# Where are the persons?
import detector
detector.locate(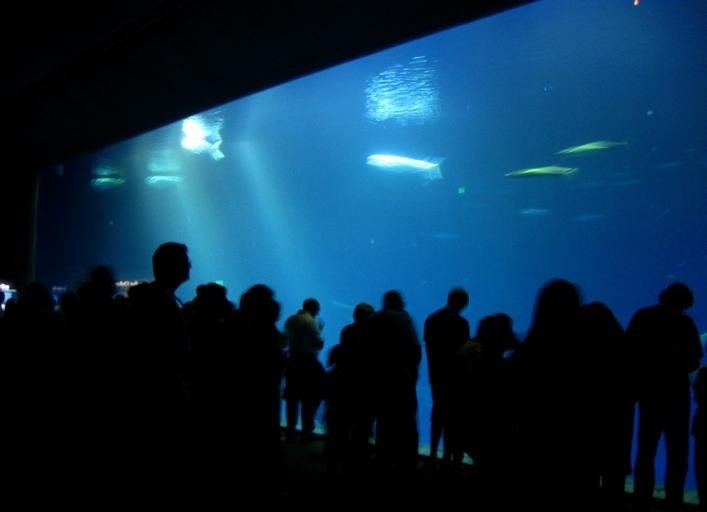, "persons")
[1,242,706,512]
